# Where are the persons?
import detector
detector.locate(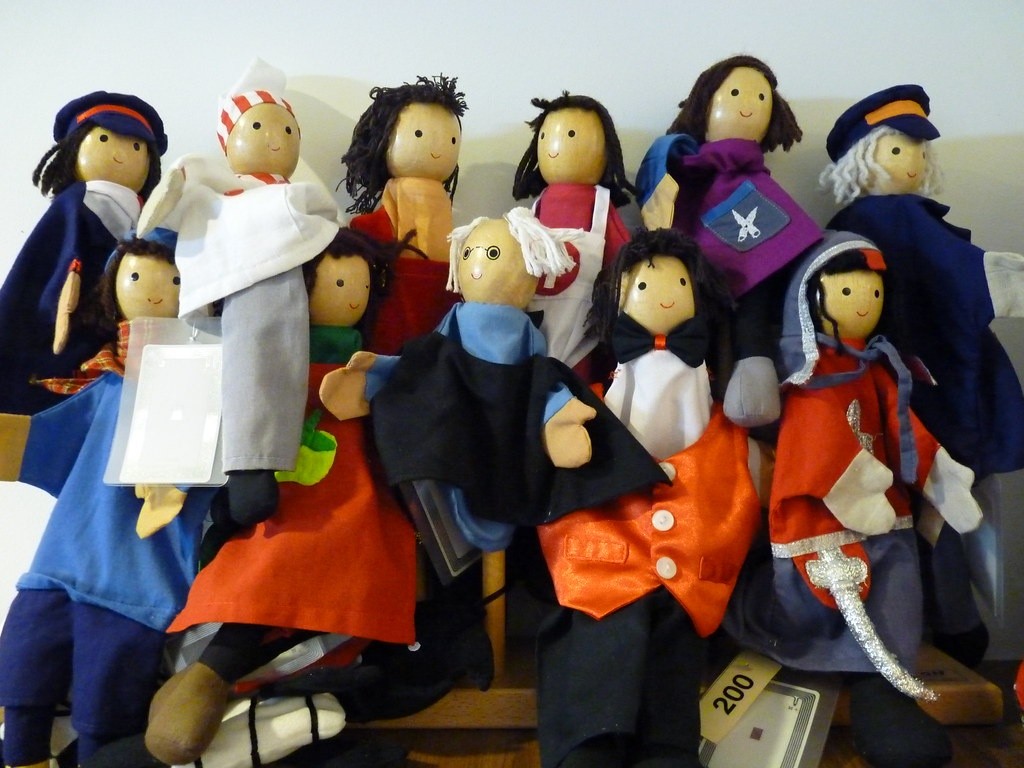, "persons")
[0,55,1024,768]
